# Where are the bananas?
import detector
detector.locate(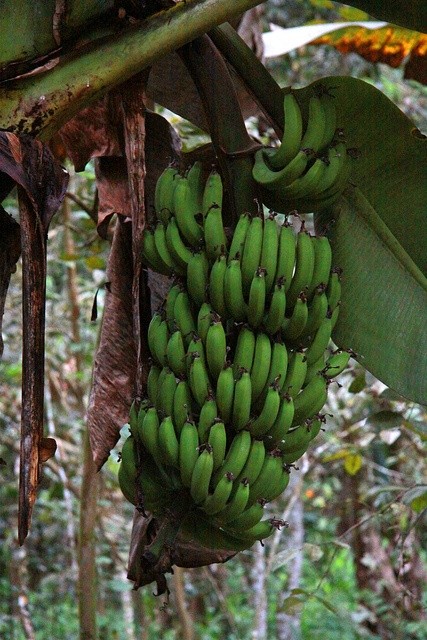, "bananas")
[117,87,353,563]
